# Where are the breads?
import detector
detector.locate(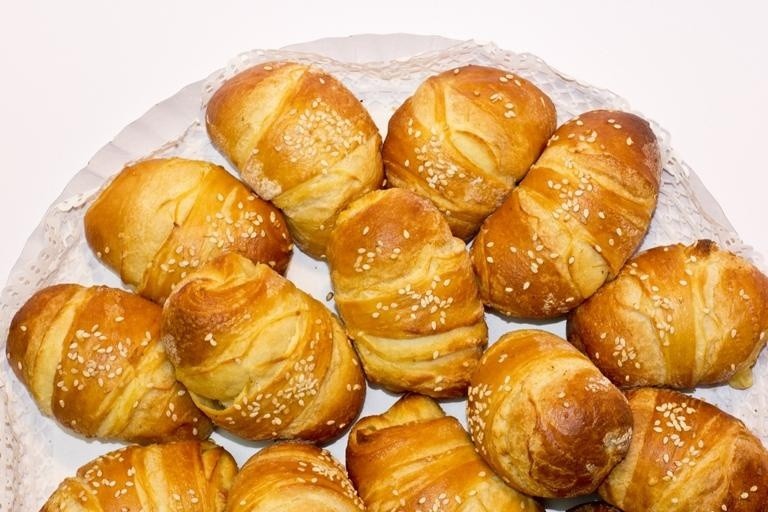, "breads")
[597,387,768,511]
[39,438,239,510]
[381,64,556,243]
[6,282,217,445]
[206,62,384,261]
[159,253,367,447]
[464,329,633,500]
[327,189,489,400]
[225,440,365,509]
[85,158,294,307]
[468,110,658,318]
[346,392,546,511]
[565,238,767,391]
